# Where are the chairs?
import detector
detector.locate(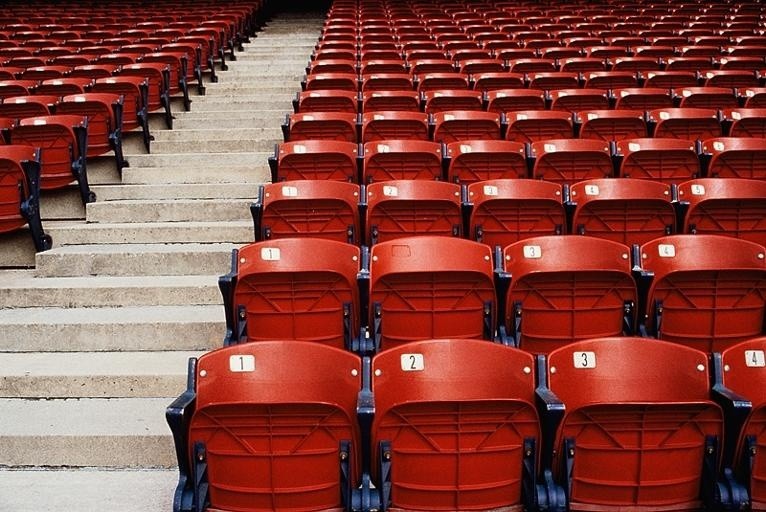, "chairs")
[164,2,765,511]
[0,0,272,255]
